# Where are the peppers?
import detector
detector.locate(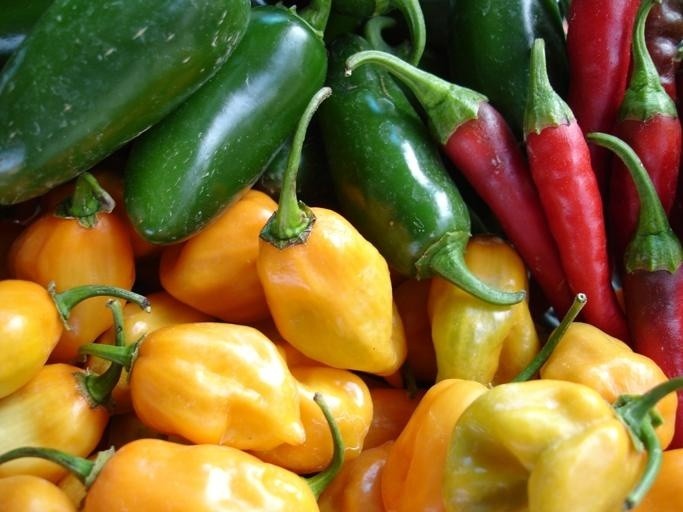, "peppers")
[0,0,682,511]
